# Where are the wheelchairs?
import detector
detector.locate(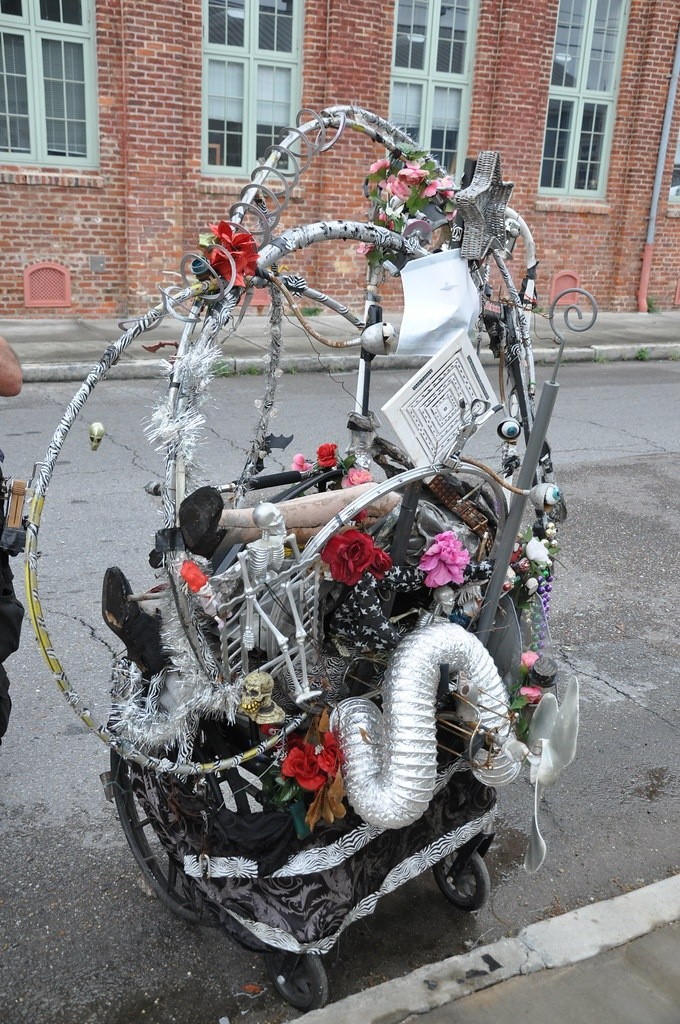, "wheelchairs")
[97,465,509,1014]
